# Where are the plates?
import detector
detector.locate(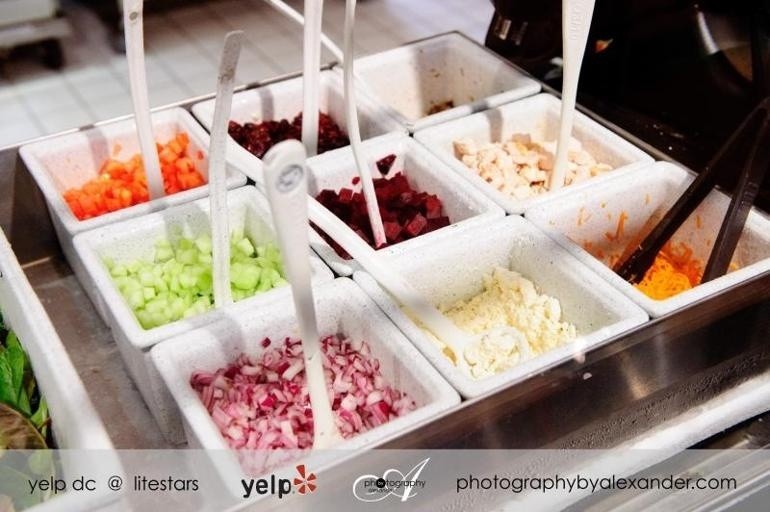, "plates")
[20,26,770,508]
